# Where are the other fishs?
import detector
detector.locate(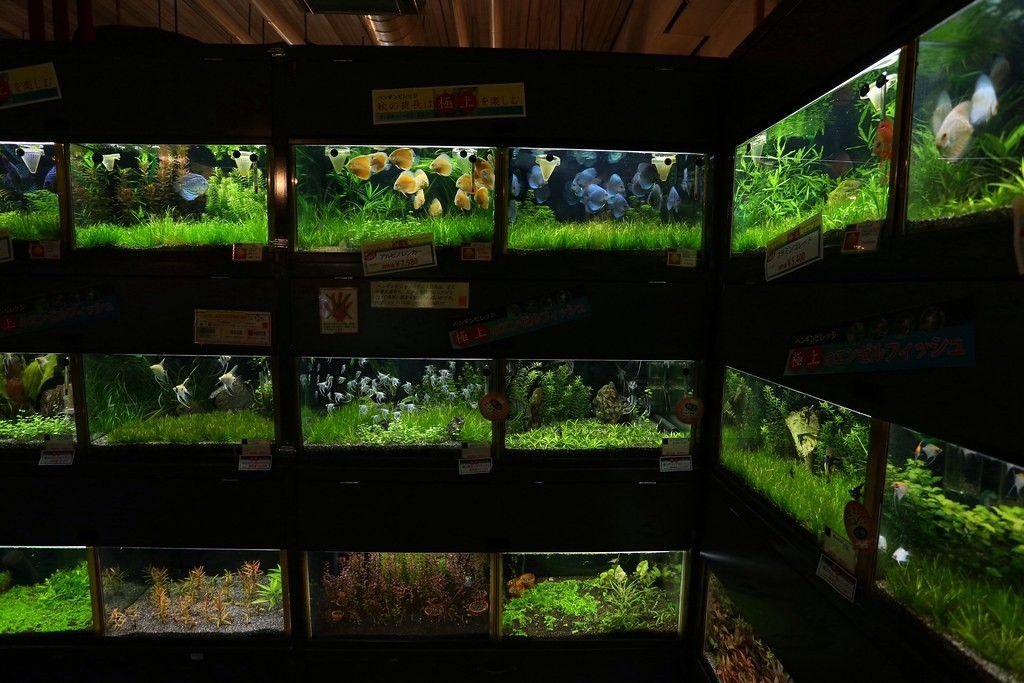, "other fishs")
[509,361,645,440]
[728,369,869,542]
[341,145,496,218]
[0,353,48,409]
[147,356,246,409]
[925,73,1000,164]
[877,433,1024,576]
[0,143,58,212]
[301,358,490,423]
[870,119,894,169]
[170,172,209,201]
[508,147,694,226]
